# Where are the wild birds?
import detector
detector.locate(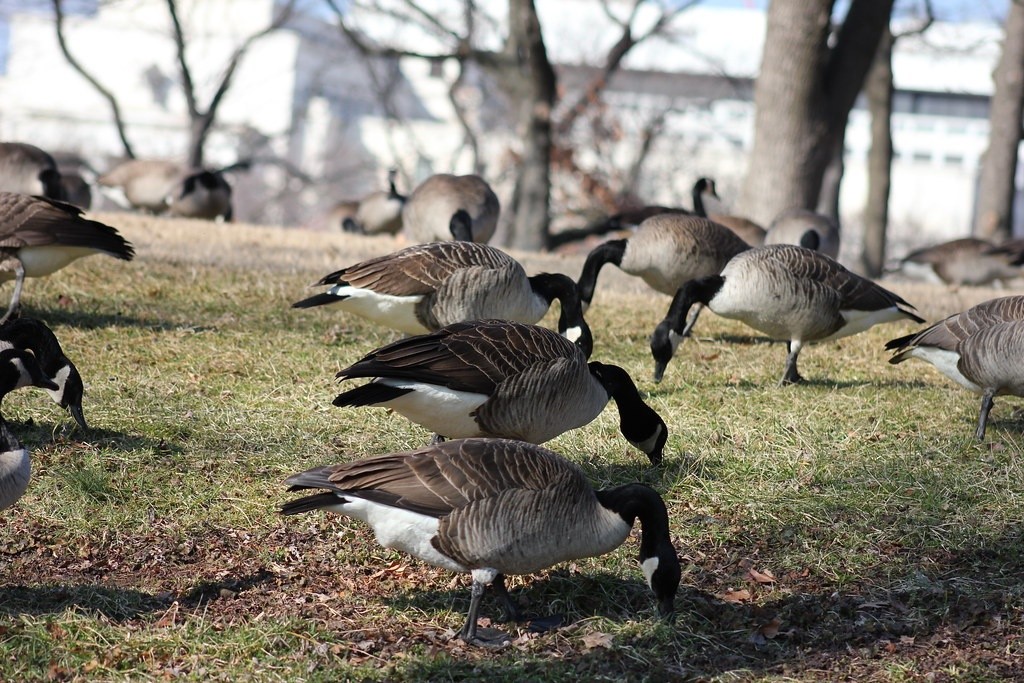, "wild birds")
[280,438,682,653]
[333,318,668,466]
[0,142,234,222]
[883,238,1024,294]
[331,170,410,236]
[404,175,501,244]
[886,295,1024,440]
[546,176,767,252]
[761,209,840,262]
[577,213,755,337]
[651,243,926,385]
[0,192,137,512]
[291,241,594,362]
